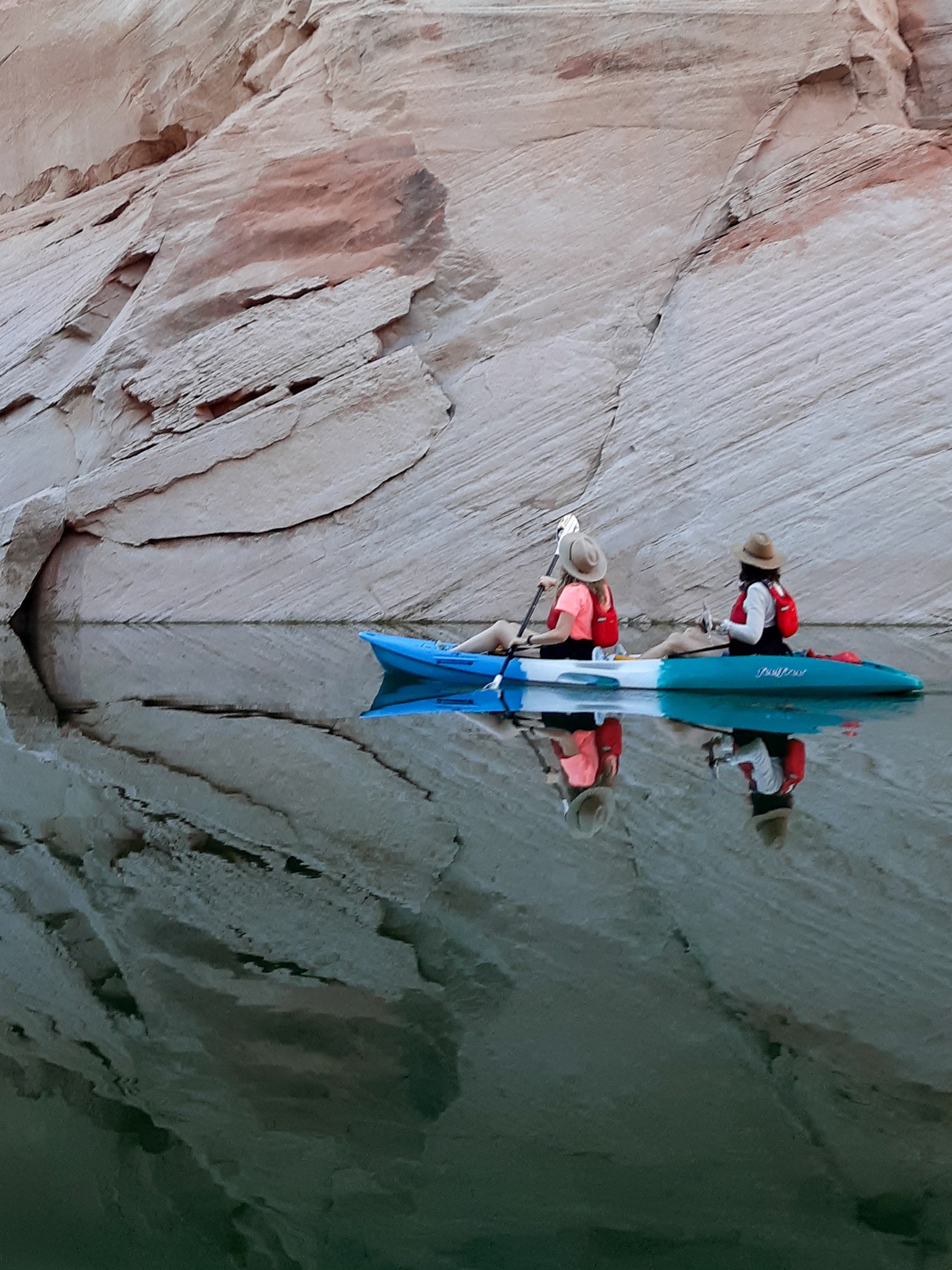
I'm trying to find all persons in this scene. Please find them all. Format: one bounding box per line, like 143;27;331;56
592;531;798;661
652;717;806;852
422;532;619;661
455;711;624;840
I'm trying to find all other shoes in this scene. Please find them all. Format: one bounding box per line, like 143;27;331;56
435;639;442;649
421;643;430;648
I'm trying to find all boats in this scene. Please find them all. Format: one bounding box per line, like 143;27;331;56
356;629;925;698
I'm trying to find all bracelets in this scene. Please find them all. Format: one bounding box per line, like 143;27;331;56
529;726;534;737
527;635;533;646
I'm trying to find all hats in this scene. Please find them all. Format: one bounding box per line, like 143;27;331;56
559;533;607;582
567;786;615;841
742;809;795;850
729;532;786;570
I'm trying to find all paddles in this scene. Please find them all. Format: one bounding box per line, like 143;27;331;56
695;600;714;635
480;512;580;698
498;690;570;823
708;734;720;779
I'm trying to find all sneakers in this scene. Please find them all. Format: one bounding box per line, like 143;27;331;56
614;644;628;658
592;646;611;661
